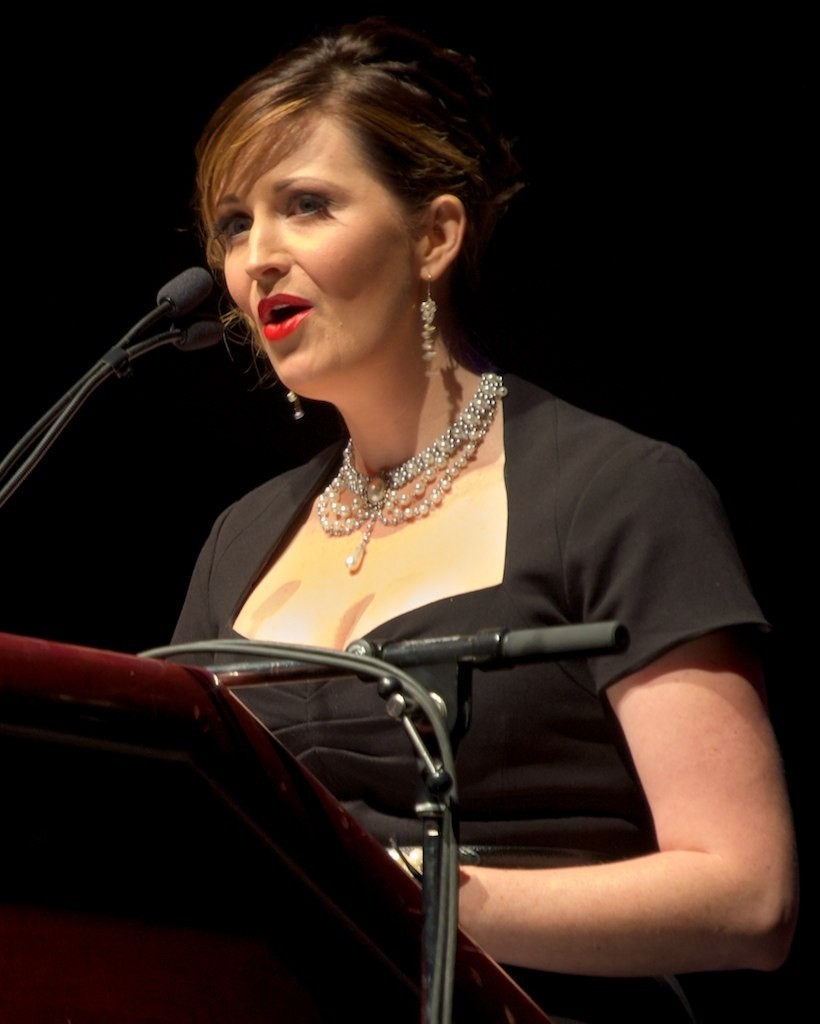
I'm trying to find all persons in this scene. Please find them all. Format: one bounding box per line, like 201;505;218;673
159;31;795;1024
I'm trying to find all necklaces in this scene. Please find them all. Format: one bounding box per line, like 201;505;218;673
315;368;508;571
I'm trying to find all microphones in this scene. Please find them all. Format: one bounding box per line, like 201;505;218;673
0;268;225;509
